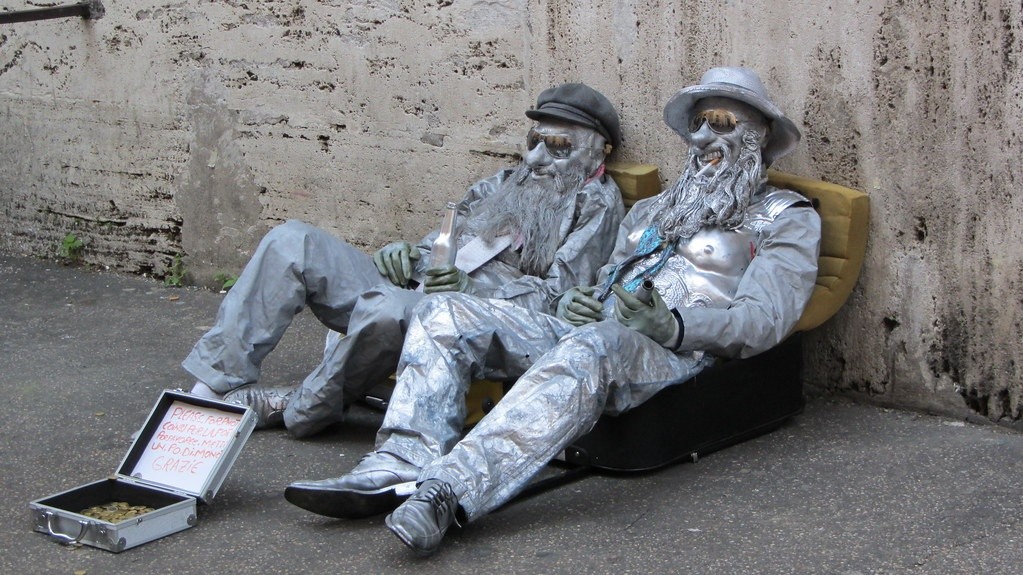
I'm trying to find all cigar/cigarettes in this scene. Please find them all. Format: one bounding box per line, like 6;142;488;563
693;157;720;180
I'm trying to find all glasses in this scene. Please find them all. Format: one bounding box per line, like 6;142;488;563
525;129;602;162
686;108;763;135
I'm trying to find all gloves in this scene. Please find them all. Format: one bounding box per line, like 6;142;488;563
374;241;421;286
556;286;605;327
610;284;677;344
423;263;467;296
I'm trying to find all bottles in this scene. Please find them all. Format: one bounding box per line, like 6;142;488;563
633;278;656;304
427;201;459;268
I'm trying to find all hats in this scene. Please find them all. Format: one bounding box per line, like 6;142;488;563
662;67;803;166
525;84;621;149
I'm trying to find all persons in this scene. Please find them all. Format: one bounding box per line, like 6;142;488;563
129;82;625;448
284;65;824;556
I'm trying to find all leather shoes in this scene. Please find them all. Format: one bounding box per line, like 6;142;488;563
220;383;311;431
284;448;443;520
129;391;225;449
385;477;463;555
